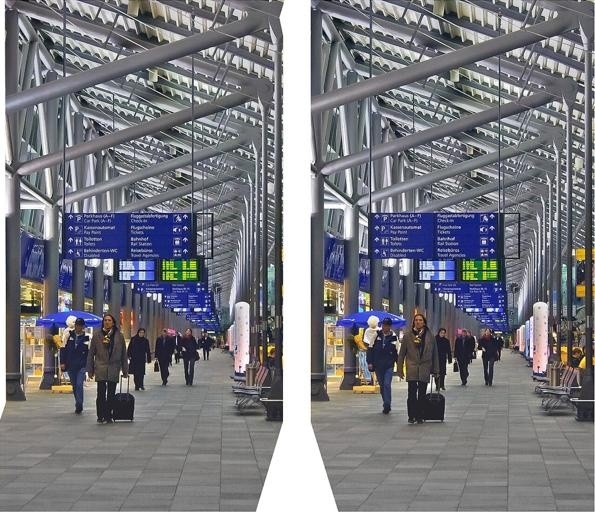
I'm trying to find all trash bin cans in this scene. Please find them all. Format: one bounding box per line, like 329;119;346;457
246;367;256;386
546;364;559;386
515;346;519;351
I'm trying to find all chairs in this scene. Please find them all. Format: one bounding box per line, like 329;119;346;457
531;359;579;415
228;361;270;414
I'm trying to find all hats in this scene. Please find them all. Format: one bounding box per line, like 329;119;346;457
383;317;392;324
74;318;84;324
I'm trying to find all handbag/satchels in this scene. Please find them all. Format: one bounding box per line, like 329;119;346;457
194;351;200;361
128;360;133;373
153;358;160;372
453;361;459;372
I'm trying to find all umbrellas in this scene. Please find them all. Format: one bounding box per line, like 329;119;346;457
36;308;103;328
336;311;407;330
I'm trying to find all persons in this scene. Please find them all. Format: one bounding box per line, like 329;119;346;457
61;314;216;424
363;315;504;423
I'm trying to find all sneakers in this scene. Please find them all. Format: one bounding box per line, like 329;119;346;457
74;409;83;414
436;386;446;391
134;386;145;391
162;380;168;386
408;415;423;424
96;416;112;423
382;407;391;414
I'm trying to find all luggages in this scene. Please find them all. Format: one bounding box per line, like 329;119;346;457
423;374;446;423
112;373;135;423
